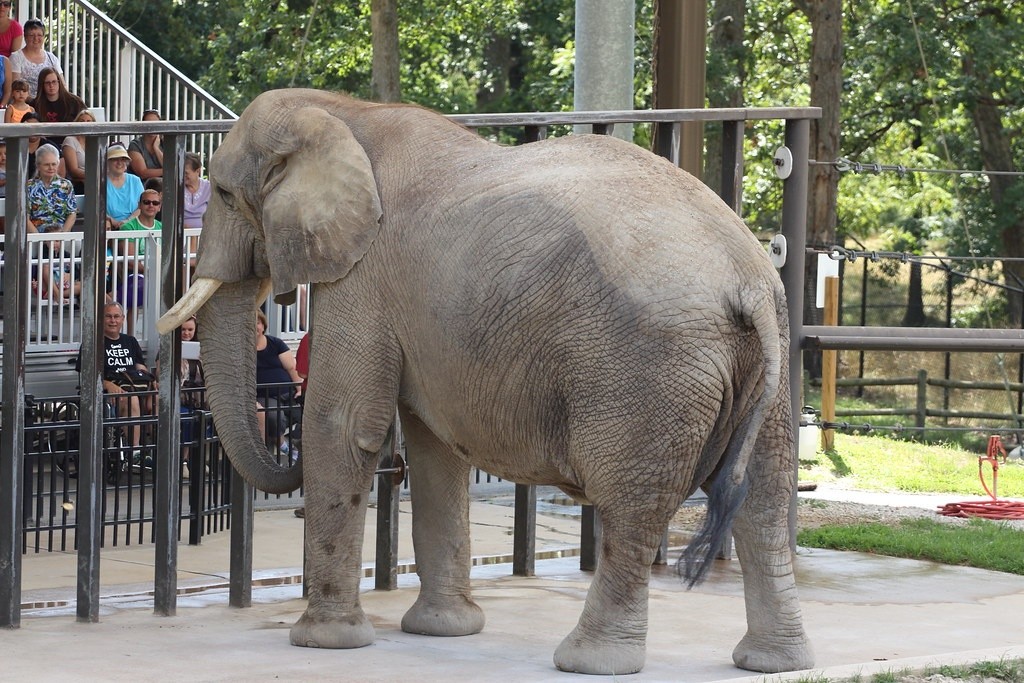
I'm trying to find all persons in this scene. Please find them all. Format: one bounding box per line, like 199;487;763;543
9;18;67;100
118;189;162;272
0;112;66;198
295;333;309;412
154;316;210;478
129;109;163;180
26;143;82;256
183;151;211;228
61;110;96;195
75;301;152;467
256;307;301;468
0;0;23;58
29;67;88;144
5;78;35;123
106;216;113;301
106;142;145;228
0;55;12;109
32;224;81;304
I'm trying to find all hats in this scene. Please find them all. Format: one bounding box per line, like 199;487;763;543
107;144;132;162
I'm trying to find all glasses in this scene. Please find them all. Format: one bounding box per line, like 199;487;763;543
44;80;59;86
145;109;159;114
141;200;160;206
104;313;124;320
0;1;12;8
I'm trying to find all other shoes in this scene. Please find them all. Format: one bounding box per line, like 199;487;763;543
182;464;190;479
295;507;305;518
134;449;153;468
290;450;299;461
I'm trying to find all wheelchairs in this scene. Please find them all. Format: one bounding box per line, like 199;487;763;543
47;357;153;484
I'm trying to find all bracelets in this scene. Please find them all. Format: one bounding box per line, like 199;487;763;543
1;103;6;108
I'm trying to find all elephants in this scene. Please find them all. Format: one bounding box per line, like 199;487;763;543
155;88;816;674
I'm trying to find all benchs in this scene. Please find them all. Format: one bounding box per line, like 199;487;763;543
0;108;107;341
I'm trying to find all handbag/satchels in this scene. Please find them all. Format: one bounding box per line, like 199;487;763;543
108;370;155;392
